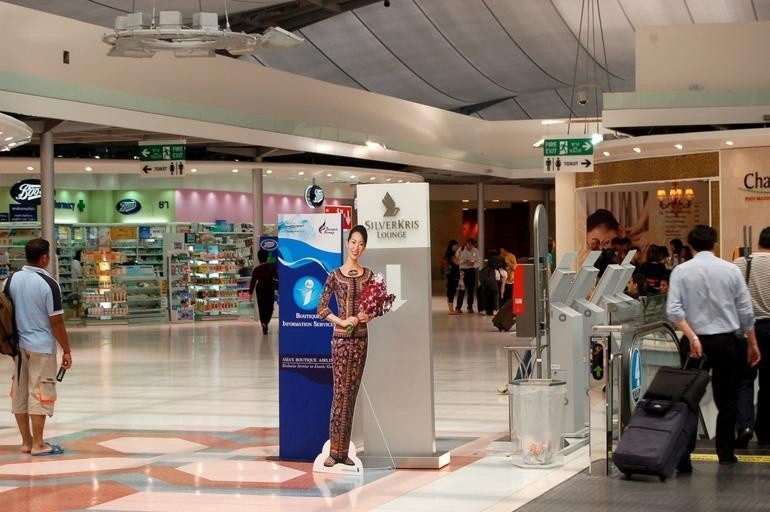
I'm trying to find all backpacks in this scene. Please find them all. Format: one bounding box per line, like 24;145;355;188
440;257;452;274
0;269;20;357
478;267;496;286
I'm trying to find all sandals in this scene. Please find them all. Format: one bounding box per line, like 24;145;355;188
324;453;355;467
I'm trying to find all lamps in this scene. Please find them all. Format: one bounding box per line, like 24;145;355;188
656;156;693;218
534;1;631;152
102;1;304;62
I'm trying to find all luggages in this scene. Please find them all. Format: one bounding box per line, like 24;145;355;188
611;400;698;483
492;299;516;332
476;285;498;313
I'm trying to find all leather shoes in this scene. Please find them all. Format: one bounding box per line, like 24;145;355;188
734;426;753;449
719;455;737;465
676;453;693;472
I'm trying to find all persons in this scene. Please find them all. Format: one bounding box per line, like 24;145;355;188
592;224;695;295
440;237;517;315
1;238;72;456
626;199;652;252
585;208;648;266
664;223;761;474
730;225;769;456
317;226;373;467
71;250;82;293
248;250;278;334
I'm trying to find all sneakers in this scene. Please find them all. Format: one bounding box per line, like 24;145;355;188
261;322;269;335
455;307;463;313
467;306;474;313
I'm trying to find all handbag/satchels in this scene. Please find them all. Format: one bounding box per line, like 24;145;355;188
642;365;712;408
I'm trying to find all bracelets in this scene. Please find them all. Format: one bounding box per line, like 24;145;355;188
687;335;698;340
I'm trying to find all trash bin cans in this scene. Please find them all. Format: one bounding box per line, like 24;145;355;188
508;378;567;469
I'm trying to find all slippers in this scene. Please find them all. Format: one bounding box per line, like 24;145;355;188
448;311;460;316
23;441;64;456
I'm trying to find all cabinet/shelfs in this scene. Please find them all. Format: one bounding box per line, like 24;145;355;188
0;220;279;328
